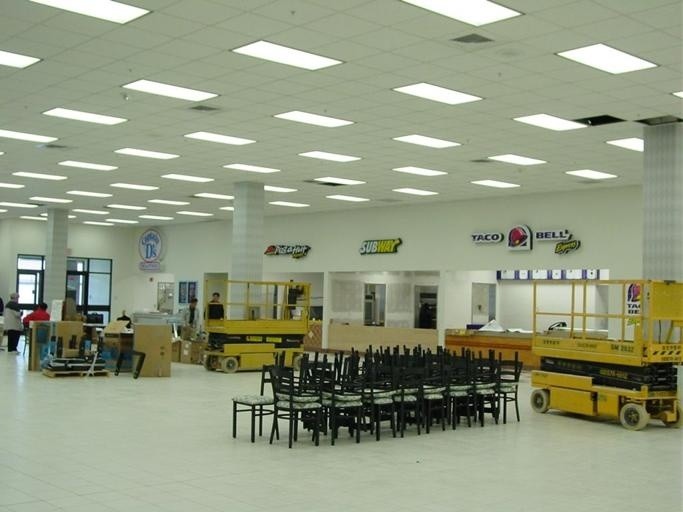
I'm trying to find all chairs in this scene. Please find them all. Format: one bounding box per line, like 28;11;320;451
20;320;30;356
228;342;523;449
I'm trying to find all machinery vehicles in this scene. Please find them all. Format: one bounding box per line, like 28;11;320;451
529;278;682;430
202;278;311;373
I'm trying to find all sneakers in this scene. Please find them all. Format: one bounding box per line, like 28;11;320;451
8;352;20;355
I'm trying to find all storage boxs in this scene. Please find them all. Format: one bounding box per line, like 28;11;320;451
57;321;82;357
180;341;206;363
134;322;171;378
30;321;60;373
171;340;179;361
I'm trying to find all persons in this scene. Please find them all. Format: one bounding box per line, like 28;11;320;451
419;302;433;328
0;296;6;350
3;292;24;355
23;302;50;328
180;298;200;341
204;292;224;320
